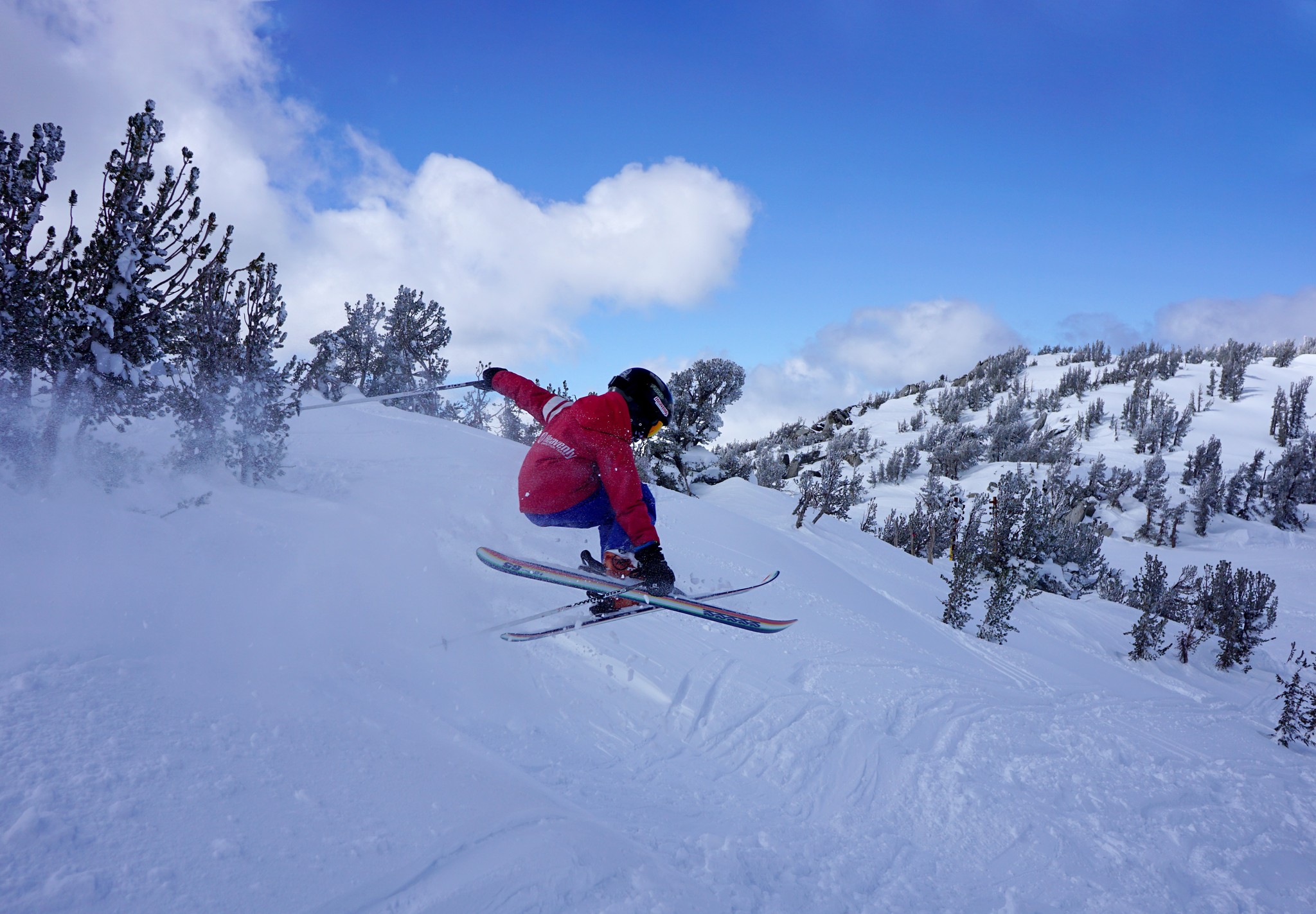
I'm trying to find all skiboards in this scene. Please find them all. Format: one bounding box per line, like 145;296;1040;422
475;546;799;643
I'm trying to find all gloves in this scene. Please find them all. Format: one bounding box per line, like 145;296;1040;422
482;367;507;387
634;544;674;597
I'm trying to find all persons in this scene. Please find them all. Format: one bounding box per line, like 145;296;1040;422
482;367;675;607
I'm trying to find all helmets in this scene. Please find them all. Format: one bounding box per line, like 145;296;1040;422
607;367;675;442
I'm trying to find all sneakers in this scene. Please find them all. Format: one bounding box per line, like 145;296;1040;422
601;550;645;582
604;593;639;610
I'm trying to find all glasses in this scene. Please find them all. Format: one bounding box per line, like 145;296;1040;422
608;375;665;440
